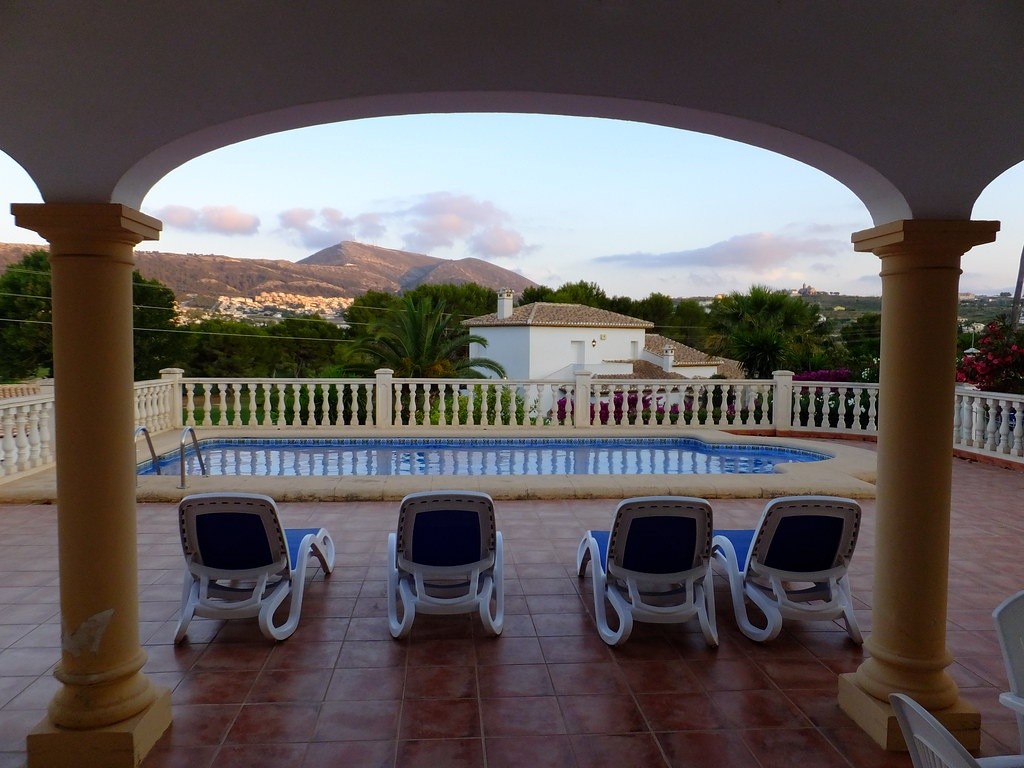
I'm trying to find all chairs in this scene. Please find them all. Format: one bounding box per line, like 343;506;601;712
387;490;504;636
173;492;335;643
889;588;1024;768
711;495;864;646
576;495;719;649
